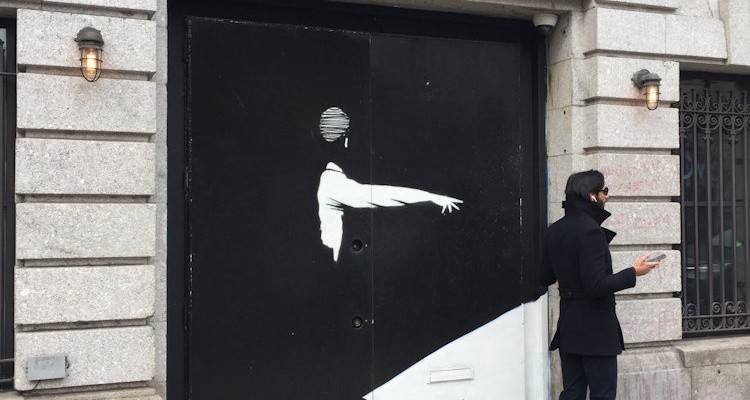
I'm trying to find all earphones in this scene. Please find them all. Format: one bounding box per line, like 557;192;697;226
591;196;597;202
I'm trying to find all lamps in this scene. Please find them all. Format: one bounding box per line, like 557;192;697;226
631;69;662;110
74;28;104;82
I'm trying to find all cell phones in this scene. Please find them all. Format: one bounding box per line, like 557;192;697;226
647;254;667;263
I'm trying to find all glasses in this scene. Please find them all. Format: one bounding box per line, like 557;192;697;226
595;187;609;195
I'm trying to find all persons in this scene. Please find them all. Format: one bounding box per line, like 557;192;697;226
542;170;660;400
